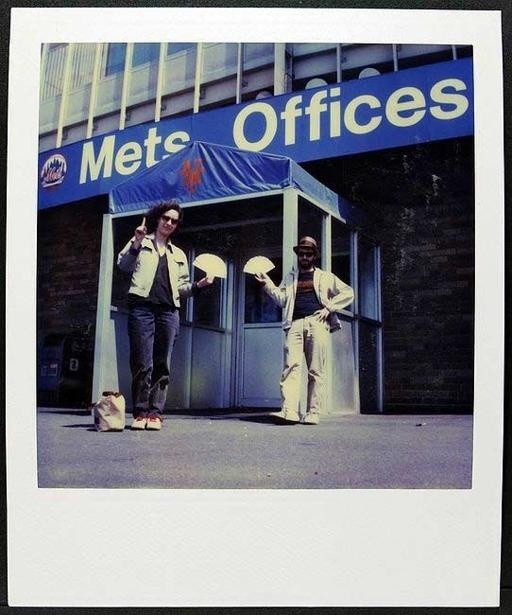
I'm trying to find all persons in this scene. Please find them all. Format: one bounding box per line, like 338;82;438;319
115;200;216;432
253;235;356;423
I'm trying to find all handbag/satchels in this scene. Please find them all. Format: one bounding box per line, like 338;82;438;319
89;390;126;432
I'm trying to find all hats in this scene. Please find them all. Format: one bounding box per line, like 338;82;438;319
163;215;178;225
293;236;319;258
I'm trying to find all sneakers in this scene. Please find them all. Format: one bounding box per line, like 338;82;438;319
131;414;161;430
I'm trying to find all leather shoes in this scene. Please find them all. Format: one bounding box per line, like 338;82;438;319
304;411;320;424
268;409;300;425
